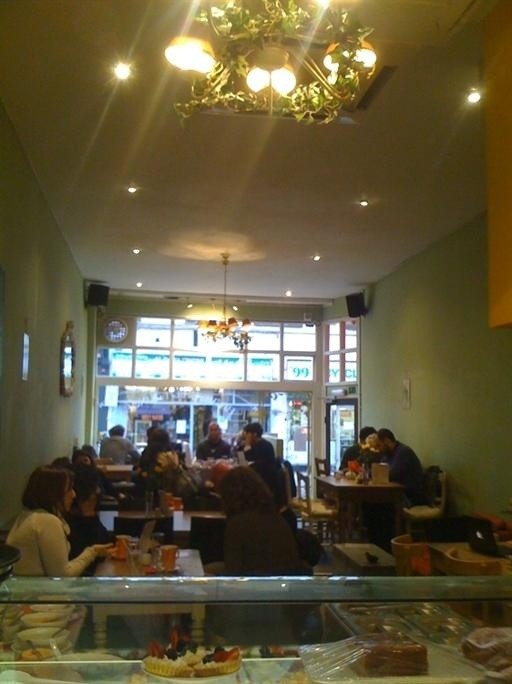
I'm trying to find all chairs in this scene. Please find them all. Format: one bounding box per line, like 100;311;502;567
94;456;505;626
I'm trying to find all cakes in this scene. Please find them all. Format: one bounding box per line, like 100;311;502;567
145;628;242;678
247;649;309;682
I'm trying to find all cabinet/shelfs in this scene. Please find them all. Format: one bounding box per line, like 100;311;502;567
1;576;512;683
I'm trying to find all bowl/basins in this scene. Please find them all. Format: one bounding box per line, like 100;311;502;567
12;602;75;660
0;545;21;585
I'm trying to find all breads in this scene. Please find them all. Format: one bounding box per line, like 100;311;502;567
347;632;430;678
463;626;511;678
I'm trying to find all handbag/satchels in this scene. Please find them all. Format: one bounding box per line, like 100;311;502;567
295;529;325;567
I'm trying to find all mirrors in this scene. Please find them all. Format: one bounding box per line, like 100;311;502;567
59;320;77;393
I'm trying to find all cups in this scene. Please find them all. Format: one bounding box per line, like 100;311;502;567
115;535;131;559
161;545;180;571
164;492;182;511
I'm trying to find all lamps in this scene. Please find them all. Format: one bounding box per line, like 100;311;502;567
196;250;255;350
163;0;377;126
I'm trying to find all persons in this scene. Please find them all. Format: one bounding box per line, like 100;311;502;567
377;428;427;509
339;427;378;473
9;420;313;639
